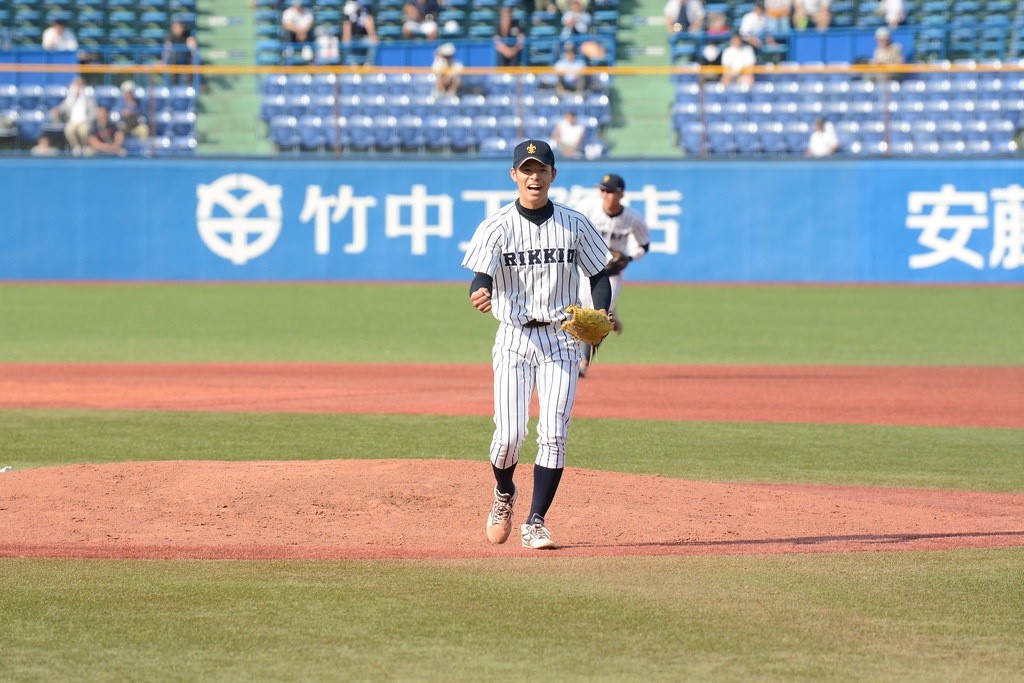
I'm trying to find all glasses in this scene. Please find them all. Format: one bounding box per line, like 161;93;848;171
601;187;614;195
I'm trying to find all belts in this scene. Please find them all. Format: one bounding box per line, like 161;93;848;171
523;318;567;329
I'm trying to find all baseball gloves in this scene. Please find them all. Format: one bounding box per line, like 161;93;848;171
555;304;616;367
606;247;628;276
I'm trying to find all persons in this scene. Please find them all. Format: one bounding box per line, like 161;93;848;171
575;172;651;378
29;16;199;158
281;0;907;97
462;141;614;549
804;115;839;158
549;108;586;162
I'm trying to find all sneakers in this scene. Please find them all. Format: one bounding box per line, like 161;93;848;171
487;480;517;545
520;512;556;550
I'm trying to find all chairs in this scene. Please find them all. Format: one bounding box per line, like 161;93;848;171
668;0;1024;160
251;0;618;162
0;0;202;157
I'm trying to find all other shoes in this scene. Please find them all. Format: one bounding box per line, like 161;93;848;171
577;359;588;378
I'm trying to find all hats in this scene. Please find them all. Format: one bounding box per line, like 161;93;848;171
594;174;625;192
513;139;554;169
